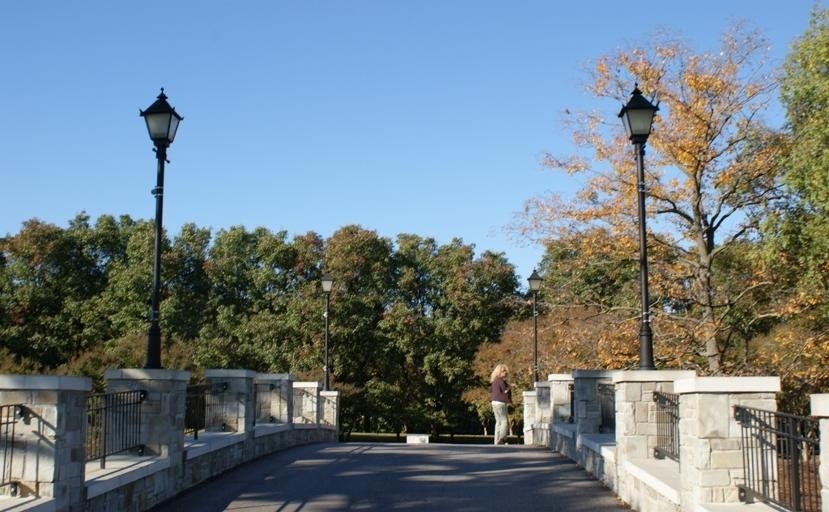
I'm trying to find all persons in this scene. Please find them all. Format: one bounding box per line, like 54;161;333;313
490;363;512;446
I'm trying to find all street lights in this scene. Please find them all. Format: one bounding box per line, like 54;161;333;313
617;79;659;370
320;270;336;391
140;86;185;368
527;267;543;381
